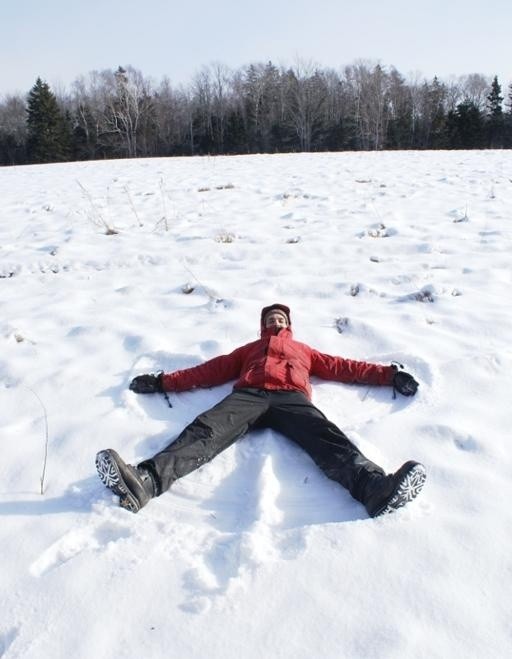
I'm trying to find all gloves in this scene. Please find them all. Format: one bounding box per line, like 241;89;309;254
130;374;163;393
393;371;419;396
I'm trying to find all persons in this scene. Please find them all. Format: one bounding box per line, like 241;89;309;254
95;303;427;520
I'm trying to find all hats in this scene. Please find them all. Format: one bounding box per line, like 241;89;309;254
263;309;289;328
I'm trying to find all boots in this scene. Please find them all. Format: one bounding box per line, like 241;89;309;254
362;461;427;518
96;449;156;513
